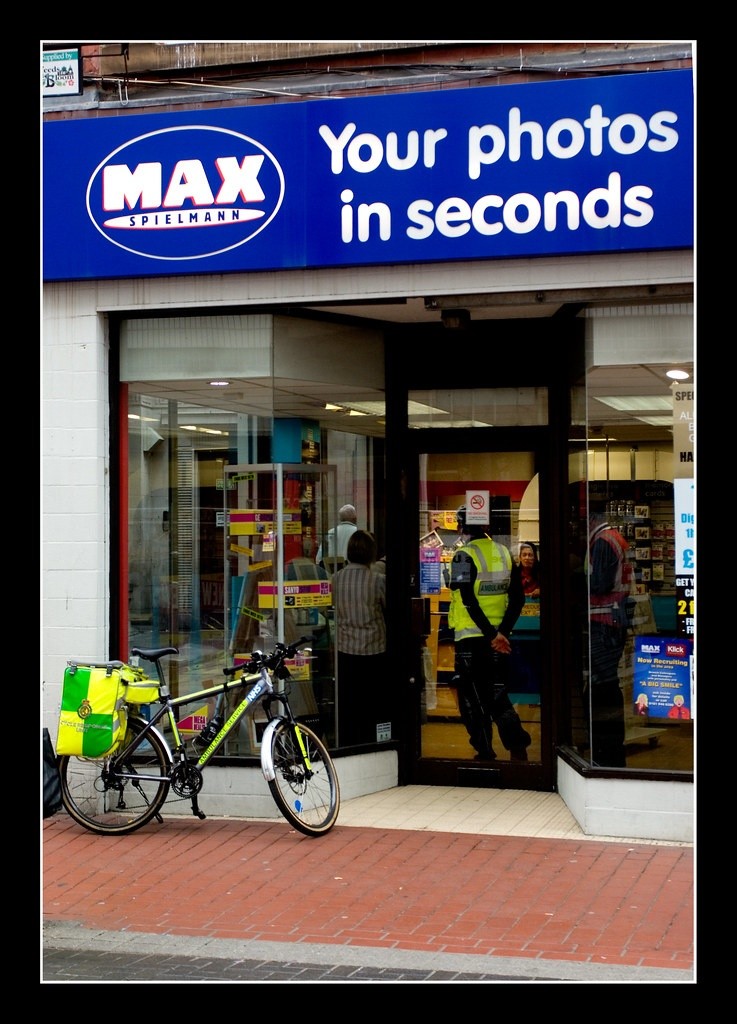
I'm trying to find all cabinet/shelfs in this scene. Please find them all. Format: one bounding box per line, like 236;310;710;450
222;462;339;758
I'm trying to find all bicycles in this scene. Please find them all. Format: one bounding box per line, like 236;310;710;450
58;635;340;837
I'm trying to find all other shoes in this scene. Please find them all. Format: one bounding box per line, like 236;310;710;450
509;748;529;762
592;750;627;768
474;748;498;761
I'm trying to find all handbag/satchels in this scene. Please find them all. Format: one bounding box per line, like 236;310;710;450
44;728;62;817
54;664;148;758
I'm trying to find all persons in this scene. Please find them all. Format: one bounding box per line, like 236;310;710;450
516;541;541;599
316;504;358;564
331;530;386;747
448;507;532;764
582;494;637;768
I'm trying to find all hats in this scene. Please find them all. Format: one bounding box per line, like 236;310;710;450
348;530;377;564
